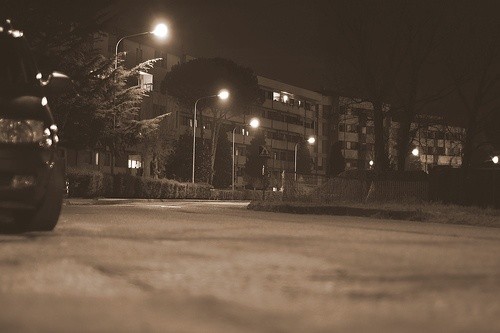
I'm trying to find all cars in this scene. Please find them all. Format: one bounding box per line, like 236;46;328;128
0;42;65;231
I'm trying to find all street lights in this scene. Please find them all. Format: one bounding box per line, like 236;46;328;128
295;136;316;182
191;88;230;185
231;117;260;191
107;20;171;199
